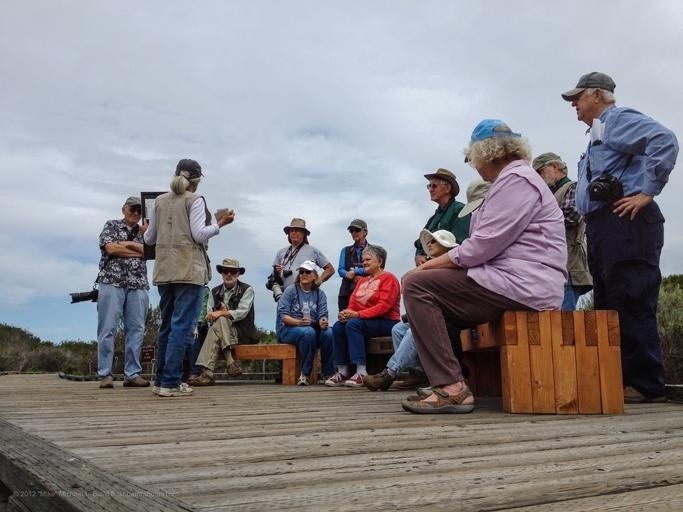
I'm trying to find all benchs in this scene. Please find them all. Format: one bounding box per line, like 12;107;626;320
367;310;625;415
183;344;322;385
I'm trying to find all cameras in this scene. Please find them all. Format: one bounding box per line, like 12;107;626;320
414;238;423;250
265;274;282;302
69;288;98;303
283;269;292;278
589;174;624;204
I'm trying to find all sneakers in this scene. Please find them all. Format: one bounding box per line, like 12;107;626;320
186;373;215;386
363;368;393;391
151;382;194;397
123;376;150;387
227;362;242;377
100;378;114;388
297;372;312;385
316;372;368;386
623;386;665;403
400;369;427;387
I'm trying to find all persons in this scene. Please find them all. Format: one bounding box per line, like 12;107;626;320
270;218;336;384
96;197;150;388
414;168;473;266
362;229;461;392
401;119;568;414
532;153;594;311
561;72;679;404
186;257;259;387
325;219;401;387
140;159;235;397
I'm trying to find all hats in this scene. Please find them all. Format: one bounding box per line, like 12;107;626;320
457;180;491;219
284;218;310;235
348;219;367;228
175;159;201;179
465;119;521;163
532;153;560;170
216;259;245;275
561;72;616;101
420;229;460;259
424;168;459;197
126;196;142;206
296;260;321;277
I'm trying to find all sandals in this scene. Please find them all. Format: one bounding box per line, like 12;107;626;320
402;386;475;414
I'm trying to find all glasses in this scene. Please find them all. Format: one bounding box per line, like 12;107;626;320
427;184;447;189
350;228;361;233
222;268;238;275
427;239;436;245
130;207;141;215
299;270;312;274
